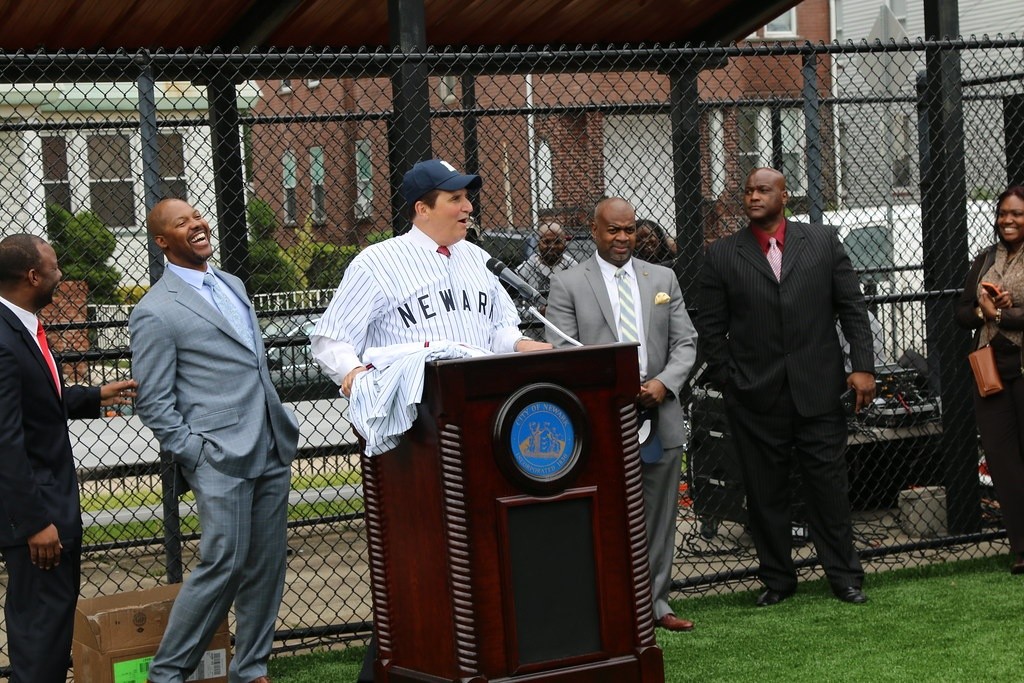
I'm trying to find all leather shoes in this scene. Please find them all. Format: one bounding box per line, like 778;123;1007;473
834;586;868;604
654;613;694;631
756;588;797;607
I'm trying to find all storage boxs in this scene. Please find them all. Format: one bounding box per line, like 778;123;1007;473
74;583;231;683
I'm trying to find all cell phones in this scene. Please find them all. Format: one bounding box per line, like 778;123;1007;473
982;282;1002;301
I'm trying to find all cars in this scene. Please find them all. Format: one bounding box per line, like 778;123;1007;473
260;312;359;402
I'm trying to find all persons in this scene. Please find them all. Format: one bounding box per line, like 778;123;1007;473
695;167;876;607
310;159;553;398
512;222;578;341
545;197;698;630
0;233;137;683
957;187;1024;573
465;216;523;307
129;198;299;683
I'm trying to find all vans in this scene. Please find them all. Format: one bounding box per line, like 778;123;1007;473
783;201;997;364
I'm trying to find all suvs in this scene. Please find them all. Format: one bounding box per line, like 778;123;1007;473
479;223;601;281
666;358;943;526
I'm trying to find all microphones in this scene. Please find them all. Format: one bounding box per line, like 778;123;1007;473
486;257;547;307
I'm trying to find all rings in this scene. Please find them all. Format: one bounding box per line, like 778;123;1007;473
120;392;123;397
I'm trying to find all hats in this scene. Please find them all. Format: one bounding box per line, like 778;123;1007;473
636;402;663;464
403;159;482;207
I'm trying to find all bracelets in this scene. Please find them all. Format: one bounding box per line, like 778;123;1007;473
994;308;1002;324
977;306;983;319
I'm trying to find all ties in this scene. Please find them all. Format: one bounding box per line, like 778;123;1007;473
615;268;643;371
766;237;782;282
37;318;60;396
203;273;256;355
437;246;451;257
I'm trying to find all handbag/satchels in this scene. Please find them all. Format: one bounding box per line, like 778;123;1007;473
966;314;1004;397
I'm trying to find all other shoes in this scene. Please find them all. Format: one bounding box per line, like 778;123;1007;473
248;675;272;683
